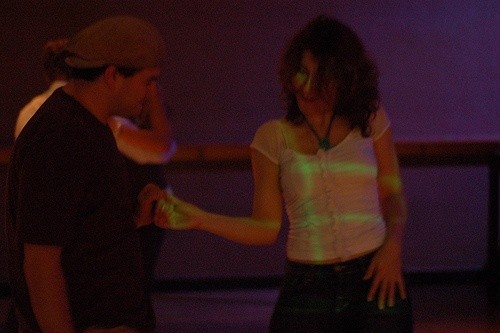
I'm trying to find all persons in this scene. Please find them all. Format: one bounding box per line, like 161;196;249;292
7;16;170;332
154;17;413;333
14;38;174;280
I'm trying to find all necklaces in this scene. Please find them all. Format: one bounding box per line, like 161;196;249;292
300;107;339;153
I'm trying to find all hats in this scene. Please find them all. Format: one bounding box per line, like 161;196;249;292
64;16;169;68
